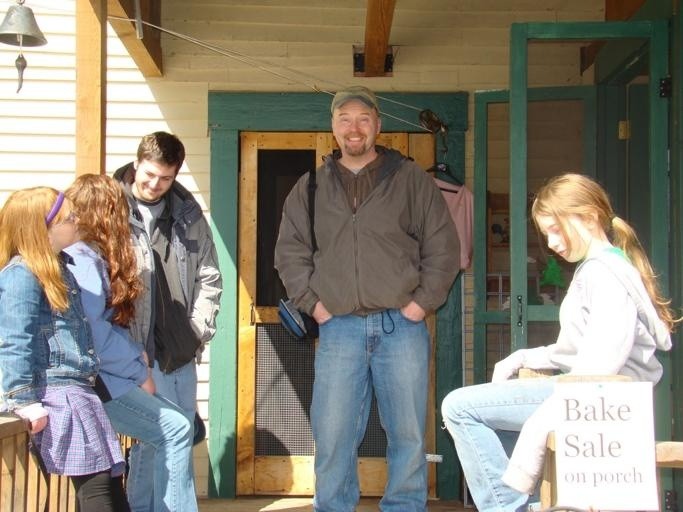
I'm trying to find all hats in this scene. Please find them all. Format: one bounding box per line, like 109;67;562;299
330;85;380;113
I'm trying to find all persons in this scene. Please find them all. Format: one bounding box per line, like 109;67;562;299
274;86;461;512
59;174;201;512
442;174;674;512
110;132;221;512
0;187;130;511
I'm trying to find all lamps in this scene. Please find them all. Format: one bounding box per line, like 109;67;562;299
0;0;48;94
